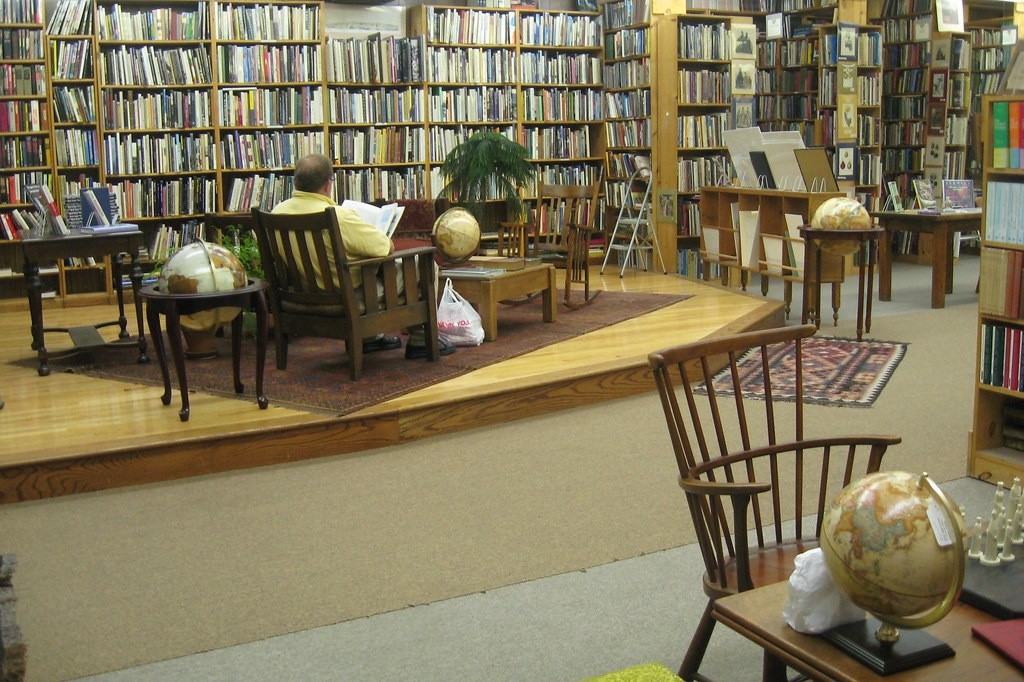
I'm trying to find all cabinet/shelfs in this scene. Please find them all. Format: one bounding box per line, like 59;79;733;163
0;0;603;310
603;0;1024;327
966;93;1024;492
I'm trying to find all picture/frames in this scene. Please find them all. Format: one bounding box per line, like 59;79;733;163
836;143;857;180
655;189;677;223
731;23;757;59
794;147;840;192
837;22;858;61
732;97;757;129
936;0;964;32
731;59;756;95
838;61;857;94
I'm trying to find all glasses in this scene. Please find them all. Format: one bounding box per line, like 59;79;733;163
332;176;335;182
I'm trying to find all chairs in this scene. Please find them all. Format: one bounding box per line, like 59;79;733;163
648;324;902;682
251;206;445;381
494;181;602;311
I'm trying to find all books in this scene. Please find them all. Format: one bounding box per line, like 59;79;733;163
0;0;324;292
677;0;1024;279
325;0;653;275
979;246;1024;451
971;618;1024;666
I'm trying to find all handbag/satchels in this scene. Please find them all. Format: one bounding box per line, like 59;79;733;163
436;276;485;345
782;545;867;635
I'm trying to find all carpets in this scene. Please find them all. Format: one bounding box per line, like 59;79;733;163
5;288;697;415
692;334;907;409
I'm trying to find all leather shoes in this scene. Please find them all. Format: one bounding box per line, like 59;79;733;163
404;338;455;358
345;335;401;354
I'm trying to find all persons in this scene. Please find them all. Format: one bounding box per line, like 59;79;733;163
266;154;456;358
958;477;1024;558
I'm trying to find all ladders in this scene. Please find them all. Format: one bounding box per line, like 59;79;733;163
600;167;668;276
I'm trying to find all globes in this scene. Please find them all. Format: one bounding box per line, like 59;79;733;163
157;240;250;331
818;469;966;677
810;197;872;256
430;206;483;269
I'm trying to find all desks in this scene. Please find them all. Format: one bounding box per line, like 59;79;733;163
868;208;982;309
437;263;558;342
12;226;148;376
711;579;1024;682
